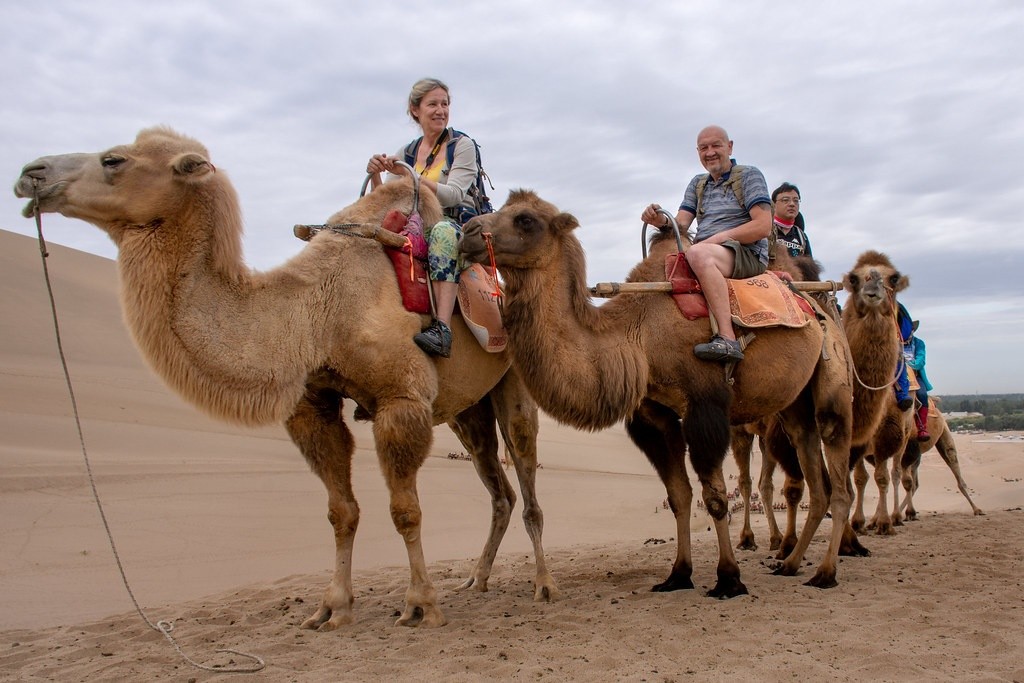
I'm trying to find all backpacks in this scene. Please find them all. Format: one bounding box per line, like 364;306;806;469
405;126;495;225
695;164;778;260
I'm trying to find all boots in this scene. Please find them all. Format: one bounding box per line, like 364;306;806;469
916;405;930;441
895;361;913;411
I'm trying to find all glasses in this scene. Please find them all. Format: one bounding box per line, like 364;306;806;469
776;197;801;204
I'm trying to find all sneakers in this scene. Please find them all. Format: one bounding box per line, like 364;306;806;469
693;334;745;361
413;319;452;358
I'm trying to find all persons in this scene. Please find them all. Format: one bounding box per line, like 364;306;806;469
771;181;819;281
641;126;772;363
895;300;912;412
365;77;478;357
905;318;933;442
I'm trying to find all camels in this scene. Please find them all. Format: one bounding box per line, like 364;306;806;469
457;189;855;601
1;125;561;632
735;250;989;560
